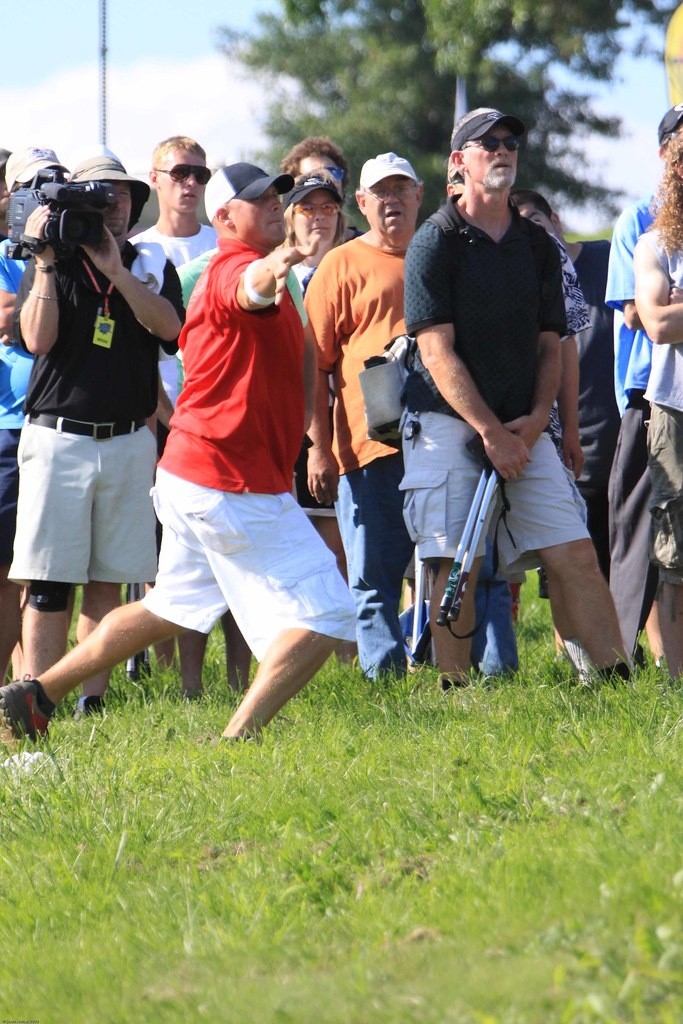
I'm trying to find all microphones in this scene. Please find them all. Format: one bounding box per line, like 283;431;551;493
40;181;69;203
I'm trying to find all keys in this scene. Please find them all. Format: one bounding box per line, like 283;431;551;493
411;421;421;449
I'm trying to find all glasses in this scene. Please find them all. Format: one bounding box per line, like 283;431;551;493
291;200;340;216
369;183;418;201
449;174;464;185
458;136;521;153
324;166;345;180
155;164;211;185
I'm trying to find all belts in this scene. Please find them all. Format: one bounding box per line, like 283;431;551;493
27;413;146;442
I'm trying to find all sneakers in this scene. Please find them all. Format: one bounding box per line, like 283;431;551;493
0;674;55;757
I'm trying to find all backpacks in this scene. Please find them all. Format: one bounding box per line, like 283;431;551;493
359;209;548;453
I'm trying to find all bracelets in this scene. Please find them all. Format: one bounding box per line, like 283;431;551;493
34;264;57;274
28;290;59;300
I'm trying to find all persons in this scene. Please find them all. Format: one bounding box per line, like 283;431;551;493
0;105;683;723
0;162;354;755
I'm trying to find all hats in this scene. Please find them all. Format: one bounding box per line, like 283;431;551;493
0;149;12;169
67;156;150;234
447;155;457;182
5;146;70;194
281;173;341;212
360;153;417;192
204;162;295;223
450;108;525;151
658;103;683;144
